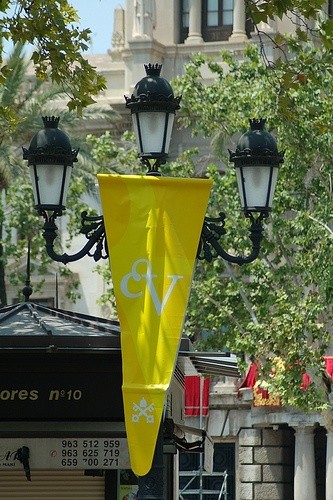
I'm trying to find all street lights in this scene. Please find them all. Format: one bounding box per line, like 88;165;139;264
19;64;287;500
